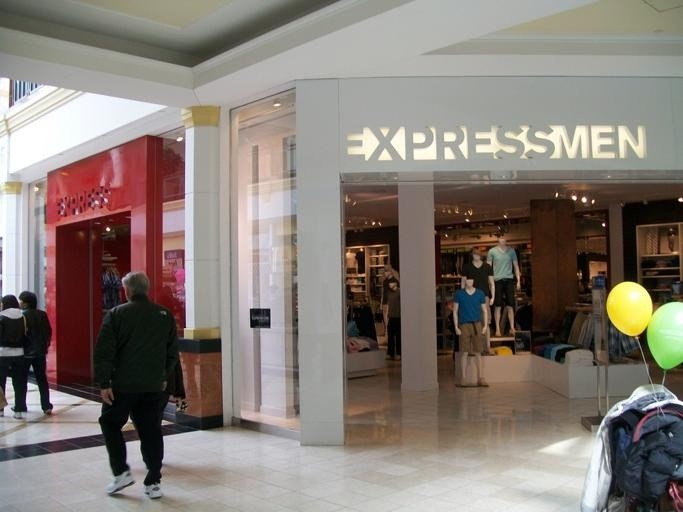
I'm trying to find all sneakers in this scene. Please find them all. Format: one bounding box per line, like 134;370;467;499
460;375;468;386
145;483;161;499
106;470;135;495
0;407;52;419
478;377;488;387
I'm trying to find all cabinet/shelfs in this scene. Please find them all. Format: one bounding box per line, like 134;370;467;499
345;246;365;305
365;244;391;302
435;285;456;354
636;222;683;313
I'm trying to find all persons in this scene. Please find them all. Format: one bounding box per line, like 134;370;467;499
381;237;521;386
0;291;53;419
93;271;186;498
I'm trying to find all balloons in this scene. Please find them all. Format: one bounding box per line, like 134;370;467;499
646;301;683;371
606;281;653;338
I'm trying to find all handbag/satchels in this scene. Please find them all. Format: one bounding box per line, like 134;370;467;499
22;314;33;353
